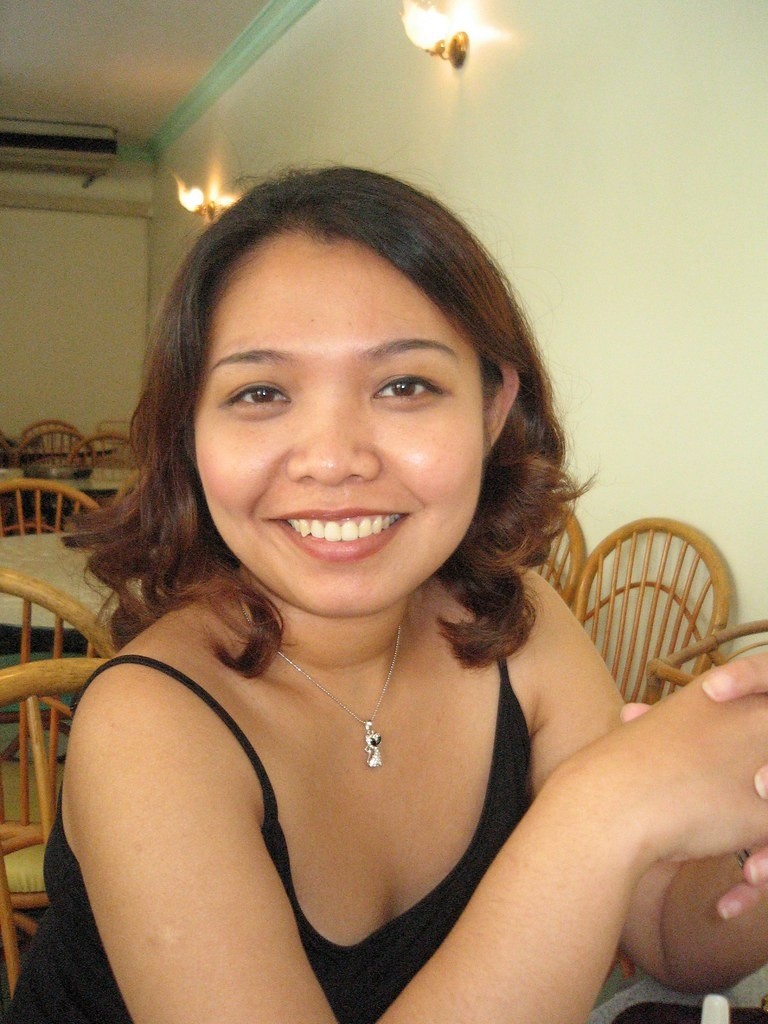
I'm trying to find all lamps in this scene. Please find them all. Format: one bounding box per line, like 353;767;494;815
403;2;471;68
175;182;223;224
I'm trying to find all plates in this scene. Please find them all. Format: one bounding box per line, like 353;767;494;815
24;468;95;481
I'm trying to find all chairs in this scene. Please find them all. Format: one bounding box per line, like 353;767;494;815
0;418;768;999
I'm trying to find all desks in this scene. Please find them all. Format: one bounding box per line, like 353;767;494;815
0;465;138;489
0;531;147;629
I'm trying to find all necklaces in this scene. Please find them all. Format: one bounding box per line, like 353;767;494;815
237;600;402;770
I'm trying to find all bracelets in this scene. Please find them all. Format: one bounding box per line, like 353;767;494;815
734;847;752;868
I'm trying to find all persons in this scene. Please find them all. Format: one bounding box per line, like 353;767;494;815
7;171;768;1024
